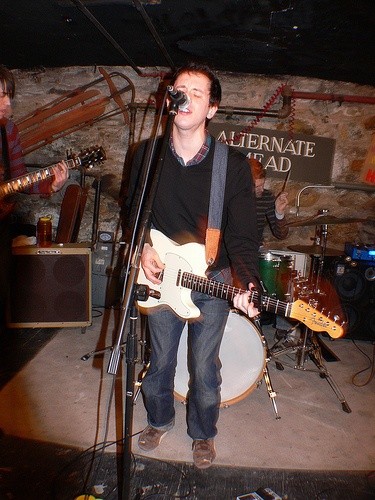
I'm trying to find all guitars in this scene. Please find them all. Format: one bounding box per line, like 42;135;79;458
135;229;346;341
0;145;107;199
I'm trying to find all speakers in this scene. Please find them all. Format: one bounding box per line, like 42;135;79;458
310;256;375;343
0;243;92;328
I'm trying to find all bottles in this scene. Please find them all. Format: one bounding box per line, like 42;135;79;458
37;216;52;247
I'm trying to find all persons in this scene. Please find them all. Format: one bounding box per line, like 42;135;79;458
119;68;260;469
0;69;70;301
247;158;290;254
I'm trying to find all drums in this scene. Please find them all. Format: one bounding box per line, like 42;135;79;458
257;251;294;303
170;264;267;409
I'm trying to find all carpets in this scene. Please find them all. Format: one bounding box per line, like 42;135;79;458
0;306;375;473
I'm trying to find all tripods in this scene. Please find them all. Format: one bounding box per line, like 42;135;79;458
81;306;151;363
268;238;352;414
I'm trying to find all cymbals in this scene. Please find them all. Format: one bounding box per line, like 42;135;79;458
286;244;345;256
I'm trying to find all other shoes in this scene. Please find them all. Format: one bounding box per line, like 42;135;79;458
138;425;168;451
191;438;216;469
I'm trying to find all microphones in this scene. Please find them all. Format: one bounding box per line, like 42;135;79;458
168;86;190;107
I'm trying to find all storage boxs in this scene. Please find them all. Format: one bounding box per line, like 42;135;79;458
345;243;375;261
